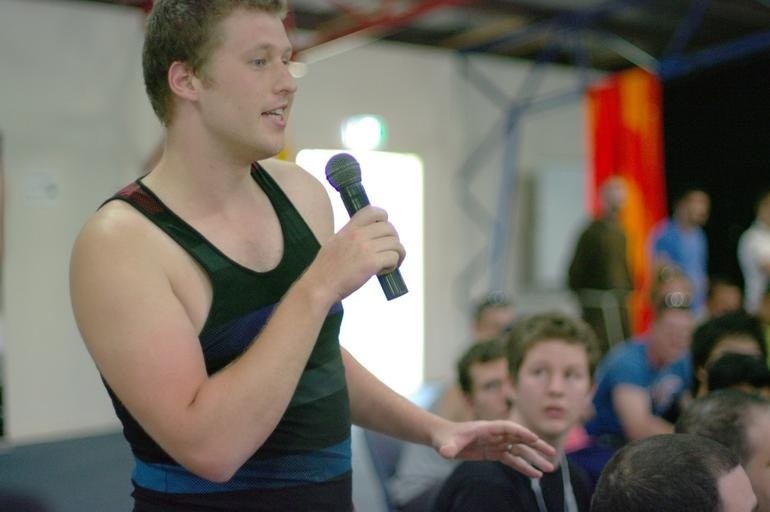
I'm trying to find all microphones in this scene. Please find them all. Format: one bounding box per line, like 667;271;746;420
324;152;409;301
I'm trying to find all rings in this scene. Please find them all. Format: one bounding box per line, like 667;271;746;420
507;443;513;453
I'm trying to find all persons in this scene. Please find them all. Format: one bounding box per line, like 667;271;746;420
566;176;638;357
69;0;556;512
652;190;711;310
399;268;769;512
737;192;770;314
